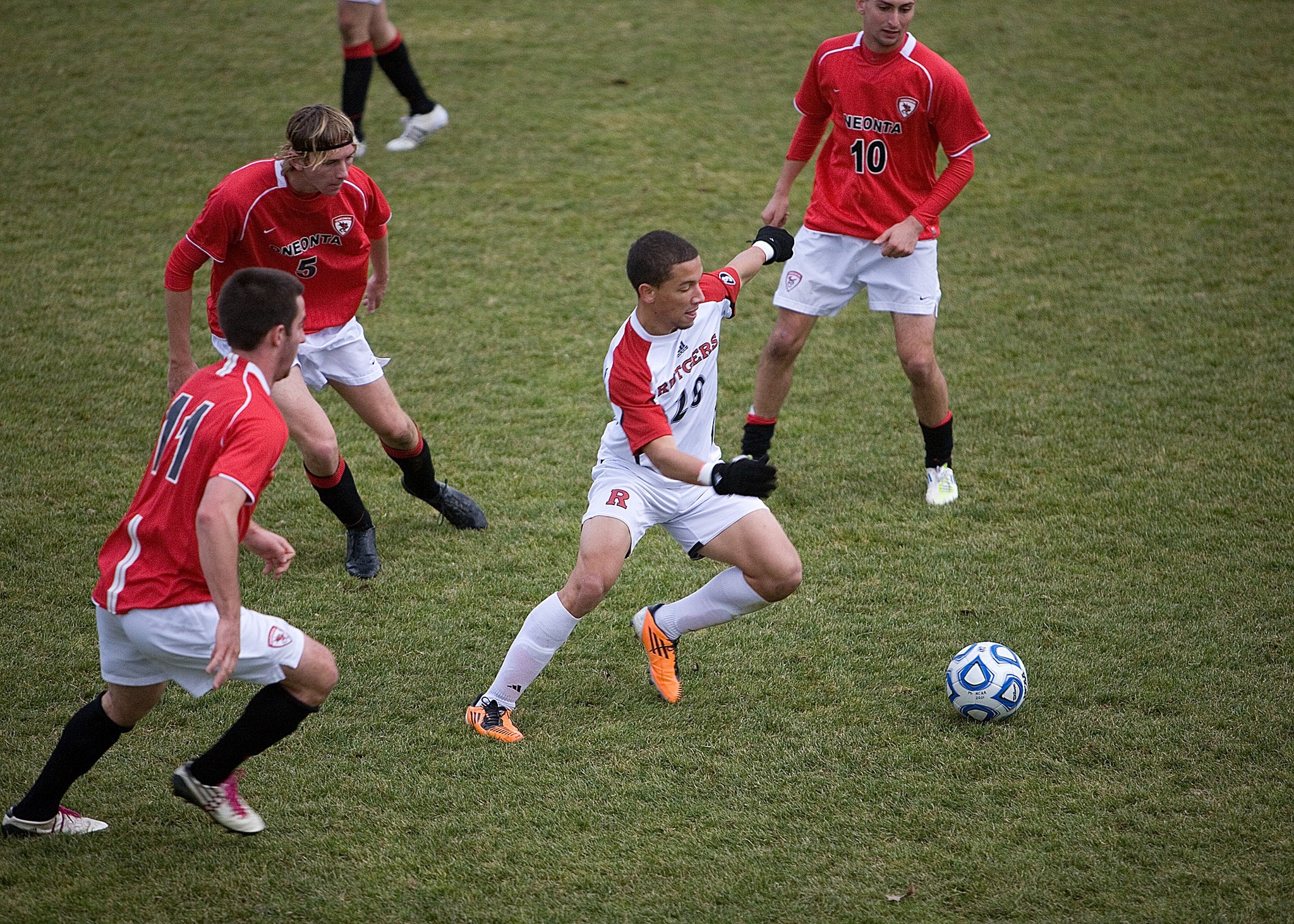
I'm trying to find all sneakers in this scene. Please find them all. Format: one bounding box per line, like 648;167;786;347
347;104;451;158
464;692;525;743
401;473;489;532
1;806;108;838
925;464;958;505
172;759;266;835
345;522;380;580
630;604;684;704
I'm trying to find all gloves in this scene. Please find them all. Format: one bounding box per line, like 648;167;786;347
748;226;797;266
697;456;779;500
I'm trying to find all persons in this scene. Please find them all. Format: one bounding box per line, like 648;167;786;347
160;104;487;580
335;0;448;163
2;265;340;838
460;222;807;742
737;0;995;508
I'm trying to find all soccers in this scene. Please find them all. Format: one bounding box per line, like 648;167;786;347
945;641;1028;723
